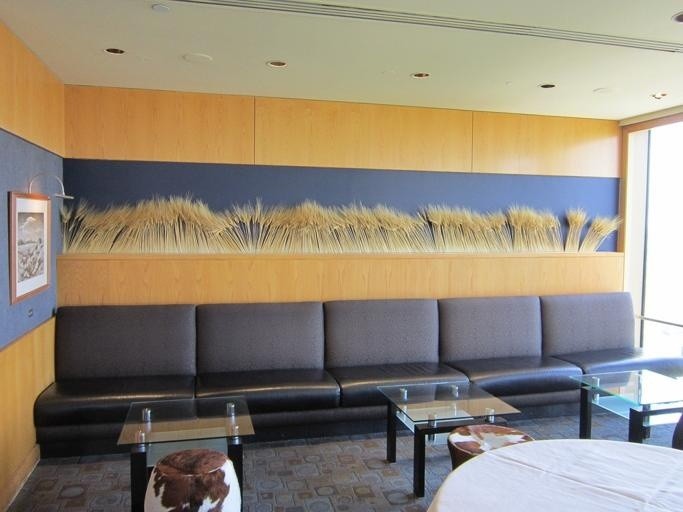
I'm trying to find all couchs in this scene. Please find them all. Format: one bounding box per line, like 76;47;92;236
34;293;683;463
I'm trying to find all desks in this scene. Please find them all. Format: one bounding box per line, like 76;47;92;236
430;440;683;511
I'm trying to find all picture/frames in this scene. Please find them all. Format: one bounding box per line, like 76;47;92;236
7;191;51;303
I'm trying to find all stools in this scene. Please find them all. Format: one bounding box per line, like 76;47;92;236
443;422;533;469
143;450;241;511
